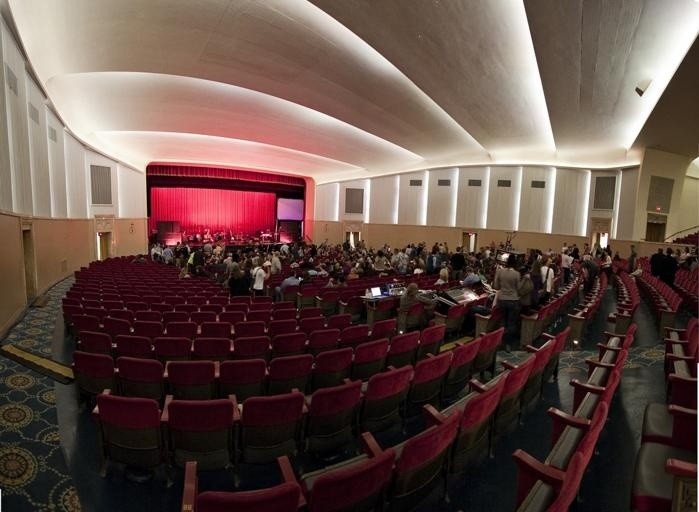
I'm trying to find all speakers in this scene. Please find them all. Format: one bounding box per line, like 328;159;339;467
156;221;182;246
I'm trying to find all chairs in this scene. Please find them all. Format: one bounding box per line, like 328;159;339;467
61;234;696;512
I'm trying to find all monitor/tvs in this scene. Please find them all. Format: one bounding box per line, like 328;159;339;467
370;286;382;297
